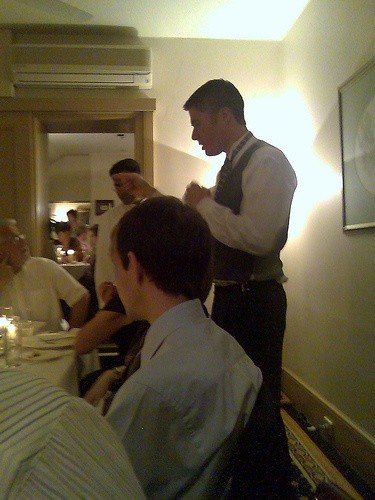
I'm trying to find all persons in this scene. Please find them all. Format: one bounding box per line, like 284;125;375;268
0;218;90;328
49;210;96;321
74;159;149;417
0;368;146;500
96;196;262;500
182;79;296;500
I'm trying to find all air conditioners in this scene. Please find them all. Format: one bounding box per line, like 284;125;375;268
7;43;154;90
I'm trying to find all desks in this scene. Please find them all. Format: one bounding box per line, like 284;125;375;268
16;327;101;397
57;262;91;280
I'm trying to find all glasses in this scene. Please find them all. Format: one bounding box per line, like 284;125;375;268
0;232;25;250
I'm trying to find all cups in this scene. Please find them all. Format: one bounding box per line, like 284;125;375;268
0;306;14;321
3;327;22;368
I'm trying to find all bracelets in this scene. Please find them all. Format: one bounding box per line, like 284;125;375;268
115;368;121;378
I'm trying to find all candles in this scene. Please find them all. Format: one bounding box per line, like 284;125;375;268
0;317;23;367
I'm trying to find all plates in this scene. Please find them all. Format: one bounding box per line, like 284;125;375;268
24;350;61;361
23;334;77;349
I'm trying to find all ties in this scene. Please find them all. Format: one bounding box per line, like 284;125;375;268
218;156;234;190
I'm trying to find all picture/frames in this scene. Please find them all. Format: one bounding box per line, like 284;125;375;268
336;53;375;233
